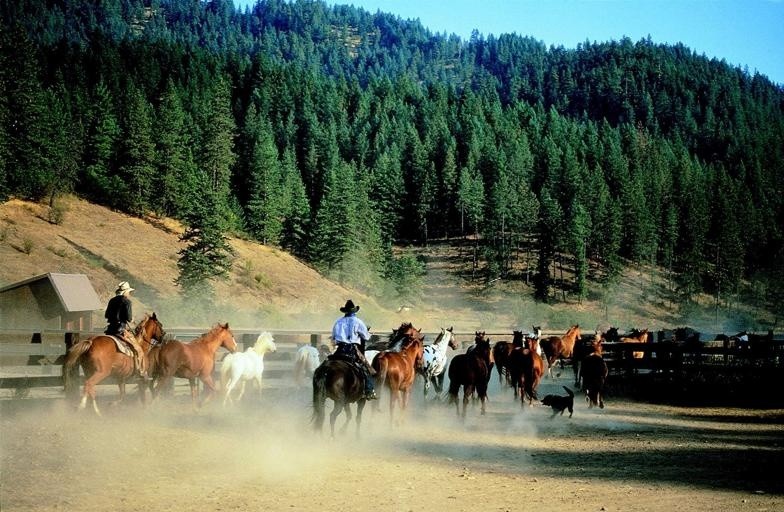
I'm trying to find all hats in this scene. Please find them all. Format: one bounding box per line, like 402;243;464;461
116;281;135;296
340;300;359;312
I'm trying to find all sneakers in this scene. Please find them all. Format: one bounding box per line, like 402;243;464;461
135;370;153;381
367;389;380;399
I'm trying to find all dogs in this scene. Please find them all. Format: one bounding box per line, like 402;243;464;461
540;385;575;418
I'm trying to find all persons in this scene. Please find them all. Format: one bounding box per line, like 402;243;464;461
332;299;379;399
104;281;151;379
737;329;749;342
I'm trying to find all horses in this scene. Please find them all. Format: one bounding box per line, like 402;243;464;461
364;322;648;428
306;327;370;443
63;313;167;419
293;343;337;387
148;322;240;416
219;332;277;409
